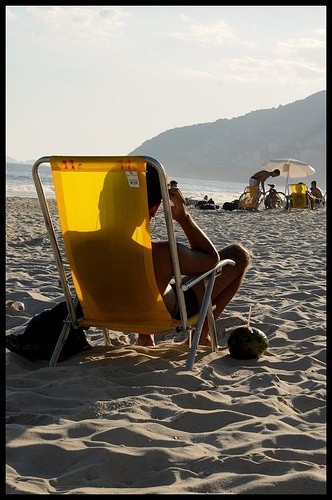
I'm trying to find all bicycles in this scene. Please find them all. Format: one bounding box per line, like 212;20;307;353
238;184;289;211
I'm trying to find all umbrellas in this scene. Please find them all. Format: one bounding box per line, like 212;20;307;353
262;157;317;203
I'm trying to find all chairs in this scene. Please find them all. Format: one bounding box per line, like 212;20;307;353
308;191;327;211
287;182;312;213
33;154;236;373
237;184;264;212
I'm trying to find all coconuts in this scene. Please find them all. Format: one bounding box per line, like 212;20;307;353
228;326;269;360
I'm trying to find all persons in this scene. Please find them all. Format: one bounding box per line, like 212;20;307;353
309;180;324;205
246;168;281;212
290;181;315;208
63;161;250;349
167;180;178;189
